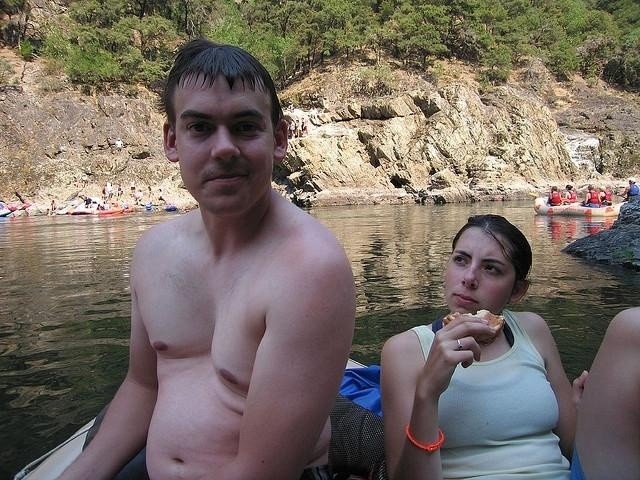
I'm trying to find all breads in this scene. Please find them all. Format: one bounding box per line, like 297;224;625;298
443;309;505;342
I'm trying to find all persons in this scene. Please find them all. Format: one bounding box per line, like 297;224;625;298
548;180;639;207
550;215;613;242
288;106;318;139
115;138;123;153
84;183;162;211
380;214;640;480
53;38;356;480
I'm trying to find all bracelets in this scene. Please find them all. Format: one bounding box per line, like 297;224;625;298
405;423;445;452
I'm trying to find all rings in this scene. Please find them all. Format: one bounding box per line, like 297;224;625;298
457;339;462;351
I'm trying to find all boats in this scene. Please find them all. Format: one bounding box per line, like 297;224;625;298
533;197;628;217
47;202;177;215
0;202;16;216
